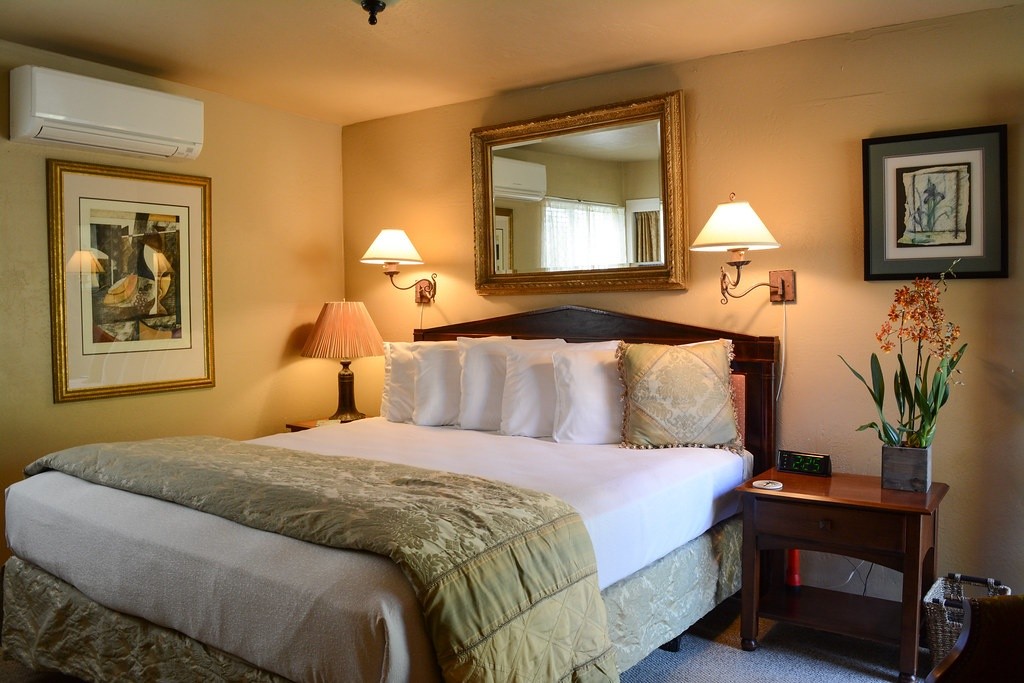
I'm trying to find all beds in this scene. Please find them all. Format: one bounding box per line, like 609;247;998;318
0;304;783;683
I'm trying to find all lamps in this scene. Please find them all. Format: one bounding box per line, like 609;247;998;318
302;299;386;420
694;190;798;303
360;229;437;303
346;0;396;25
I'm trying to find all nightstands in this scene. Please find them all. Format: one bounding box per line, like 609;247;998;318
733;466;949;683
286;417;375;432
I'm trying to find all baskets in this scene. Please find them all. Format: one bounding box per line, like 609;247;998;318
923;573;1012;670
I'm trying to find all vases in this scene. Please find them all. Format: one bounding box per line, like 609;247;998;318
878;440;939;492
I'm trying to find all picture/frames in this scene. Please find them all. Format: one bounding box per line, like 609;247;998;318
47;157;218;402
859;123;1012;281
491;206;516;277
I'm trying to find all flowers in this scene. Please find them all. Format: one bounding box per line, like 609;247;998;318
837;282;967;445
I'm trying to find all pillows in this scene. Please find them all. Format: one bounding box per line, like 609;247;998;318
456;335;511;434
551;339;627;446
377;339;420;423
498;336;567;439
615;338;743;453
406;340;463;427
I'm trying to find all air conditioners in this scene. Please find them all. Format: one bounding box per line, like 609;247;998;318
7;64;204;162
492;155;548;202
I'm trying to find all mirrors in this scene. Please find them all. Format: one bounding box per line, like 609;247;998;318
469;88;691;297
